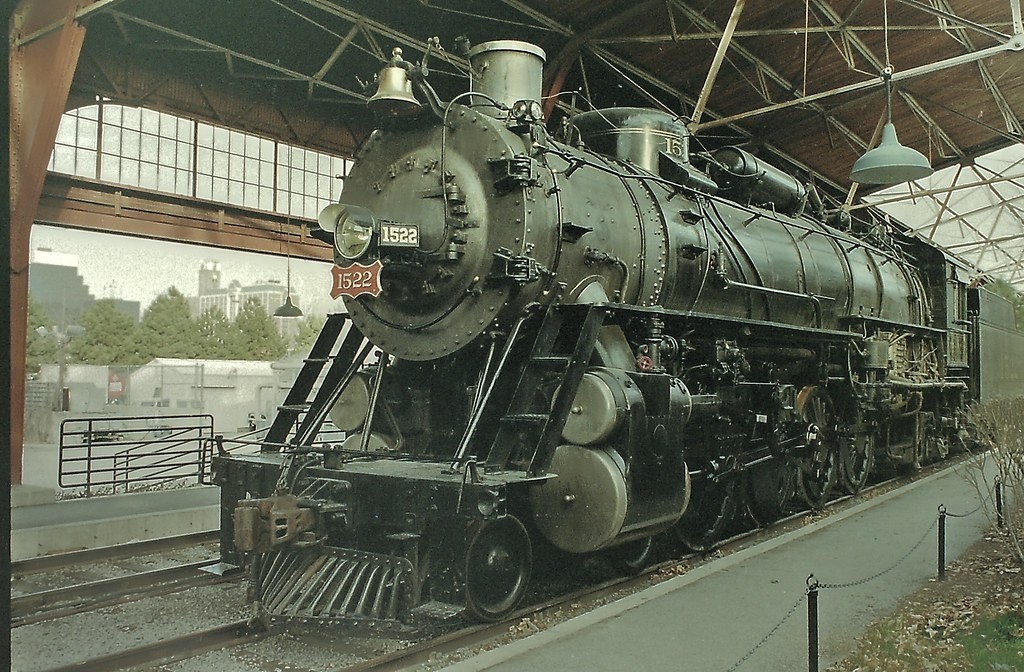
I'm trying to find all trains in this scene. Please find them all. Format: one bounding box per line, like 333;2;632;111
198;39;1024;631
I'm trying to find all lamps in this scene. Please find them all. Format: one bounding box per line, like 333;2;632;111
848;0;934;186
273;125;304;319
